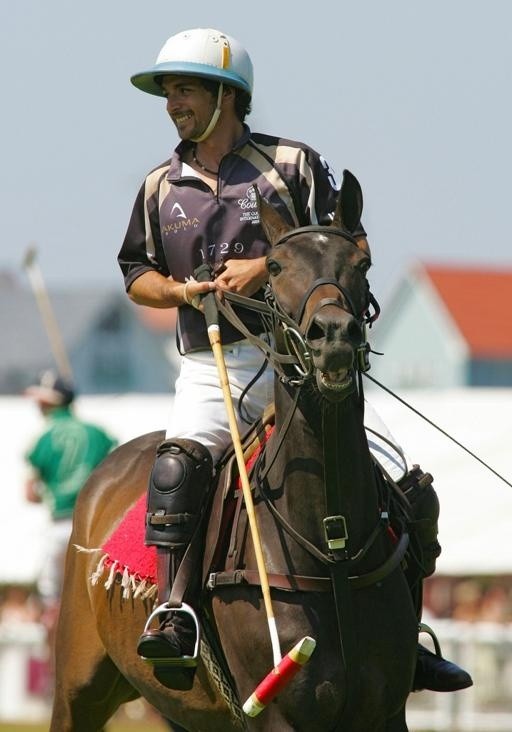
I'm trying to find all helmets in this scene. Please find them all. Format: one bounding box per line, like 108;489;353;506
130;26;256;93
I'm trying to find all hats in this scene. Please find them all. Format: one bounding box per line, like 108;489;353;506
23;374;80;409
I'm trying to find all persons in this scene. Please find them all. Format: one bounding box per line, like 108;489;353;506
110;17;475;694
18;365;120;637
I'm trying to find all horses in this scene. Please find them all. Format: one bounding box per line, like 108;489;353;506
45;168;419;732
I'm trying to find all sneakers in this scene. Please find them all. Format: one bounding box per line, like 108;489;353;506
136;621;198;692
413;639;474;692
